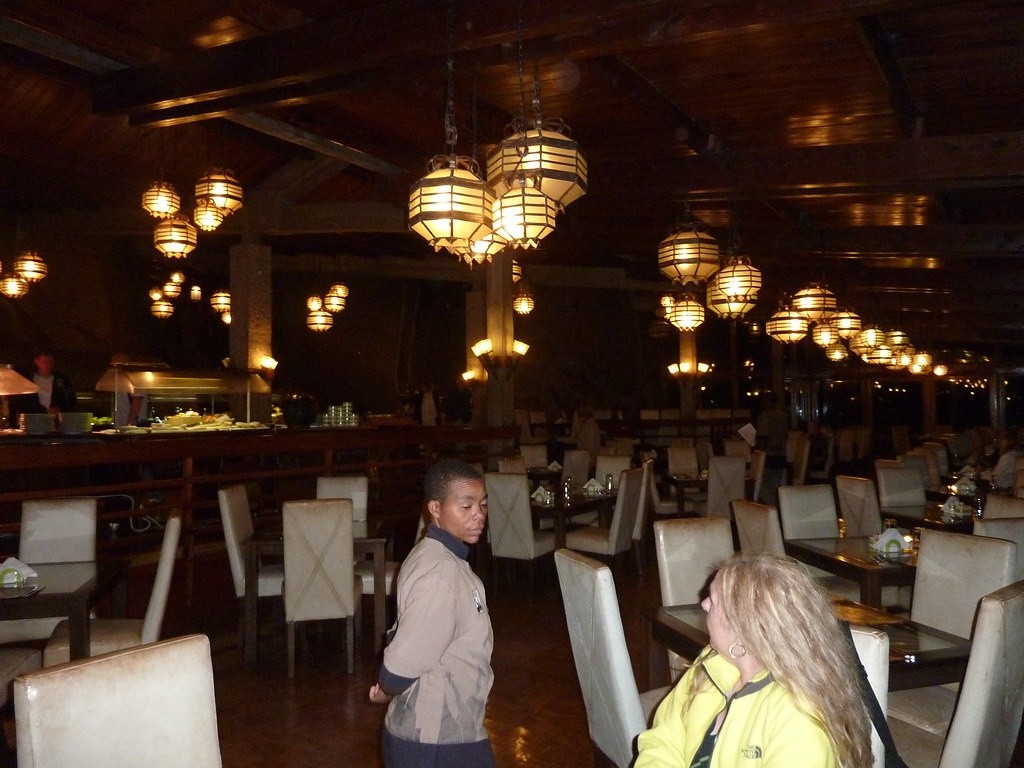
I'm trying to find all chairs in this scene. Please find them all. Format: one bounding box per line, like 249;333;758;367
0;405;1024;768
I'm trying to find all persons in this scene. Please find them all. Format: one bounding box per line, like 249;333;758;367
805;418;827;482
980;438;1024;493
414;379;475;427
18;345;78;416
369;459;497;768
112;351;148;428
629;552;878;768
545;407;602;481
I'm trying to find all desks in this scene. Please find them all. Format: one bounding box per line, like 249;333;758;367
0;421;1024;768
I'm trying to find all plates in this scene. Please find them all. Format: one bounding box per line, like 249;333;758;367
20;413;94;435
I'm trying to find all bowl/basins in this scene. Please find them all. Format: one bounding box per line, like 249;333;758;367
167;416;202;426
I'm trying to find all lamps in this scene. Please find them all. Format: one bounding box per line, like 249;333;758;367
142;118;243;260
306;248;349;332
222;357;230;367
260;355;278;380
749;322;762;335
408;0;588;272
658;153;761;333
0;249;48;299
512;253;522;283
668;363;709;383
471;339;497;379
507;340;529;381
767;282;948;376
513;288;534;314
148;269;232;325
